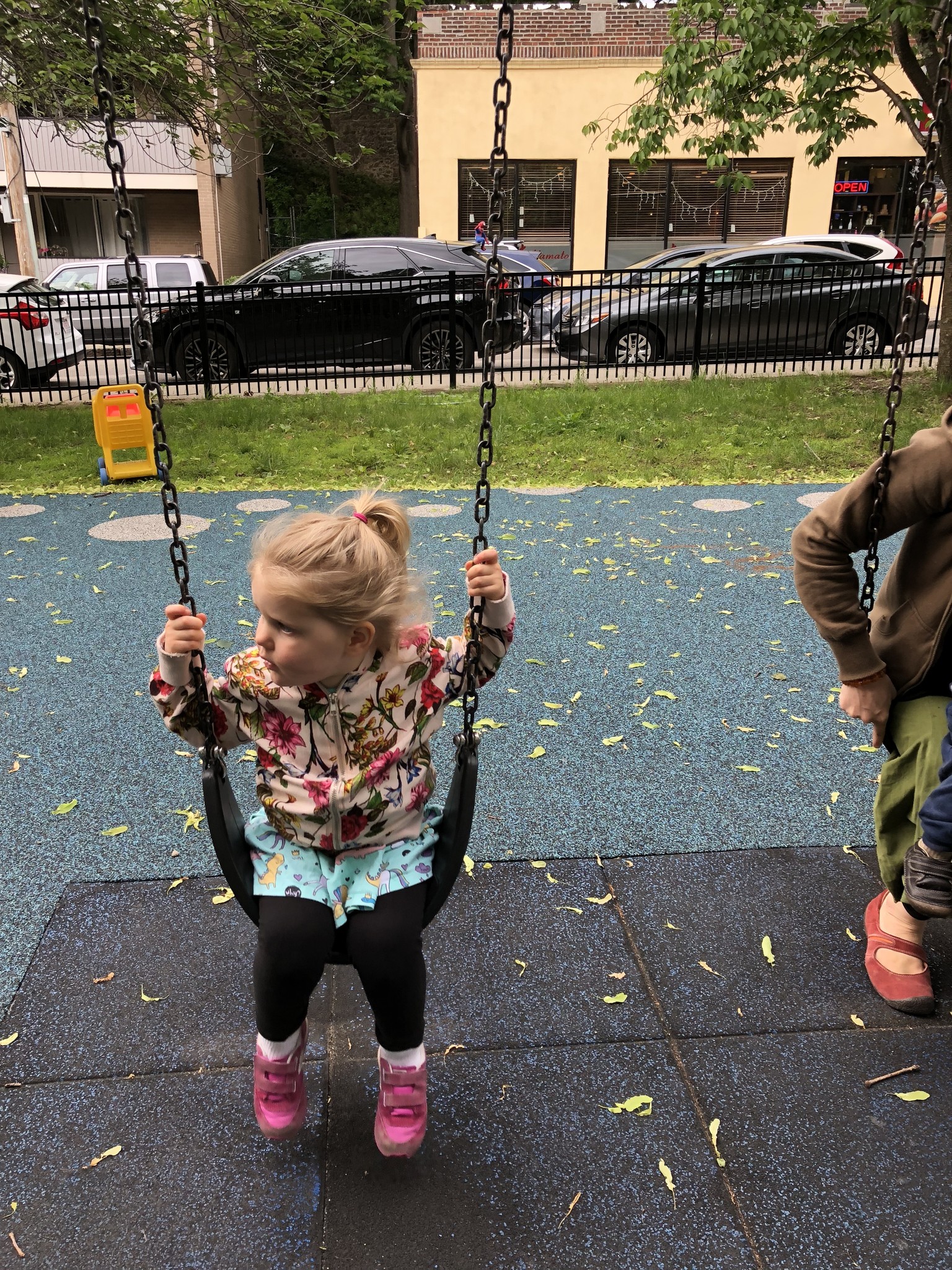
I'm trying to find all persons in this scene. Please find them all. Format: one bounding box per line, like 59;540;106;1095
150;487;513;1159
792;407;951;1010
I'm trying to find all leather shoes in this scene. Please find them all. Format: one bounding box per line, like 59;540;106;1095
904;838;952;917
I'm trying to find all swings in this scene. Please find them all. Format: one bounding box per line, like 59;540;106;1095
858;1;952;763
82;0;516;967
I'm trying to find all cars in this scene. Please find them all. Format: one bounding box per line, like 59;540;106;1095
523;243;750;347
546;244;930;367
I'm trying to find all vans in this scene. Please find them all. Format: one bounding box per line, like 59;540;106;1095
33;255;220;344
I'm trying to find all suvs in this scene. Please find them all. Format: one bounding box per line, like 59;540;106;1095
127;233;534;383
471;240;564;345
0;271;87;394
753;233;907;275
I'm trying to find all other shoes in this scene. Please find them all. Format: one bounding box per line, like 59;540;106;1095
861;889;934;1015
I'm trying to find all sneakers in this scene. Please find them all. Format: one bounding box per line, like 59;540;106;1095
252;1016;310;1140
373;1044;428;1160
